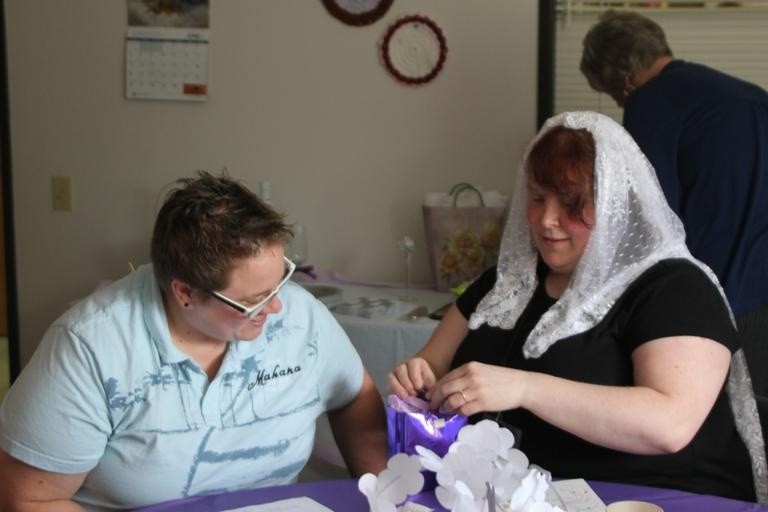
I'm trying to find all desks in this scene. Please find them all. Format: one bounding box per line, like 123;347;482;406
297;285;479;469
125;475;765;512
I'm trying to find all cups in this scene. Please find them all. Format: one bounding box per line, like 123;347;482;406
287;224;307;263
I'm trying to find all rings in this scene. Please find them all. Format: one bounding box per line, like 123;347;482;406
459;390;468;403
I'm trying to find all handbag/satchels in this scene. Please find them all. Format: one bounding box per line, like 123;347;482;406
422;183;505;292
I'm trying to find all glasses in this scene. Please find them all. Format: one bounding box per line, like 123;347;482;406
171;255;297;317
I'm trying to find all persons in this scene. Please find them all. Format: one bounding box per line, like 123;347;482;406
0;166;388;510
577;8;766;397
388;111;766;501
